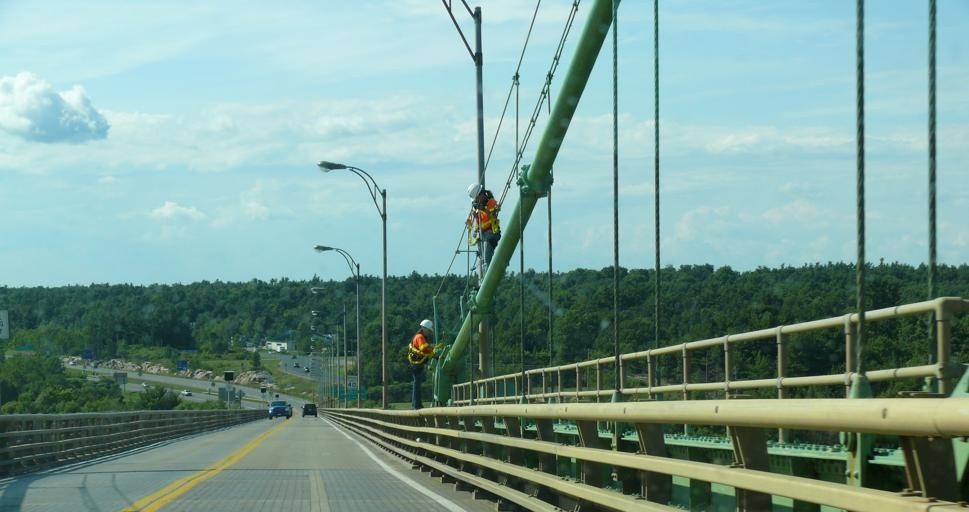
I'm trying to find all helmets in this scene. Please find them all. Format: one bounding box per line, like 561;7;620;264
467;183;483;201
419;319;433;333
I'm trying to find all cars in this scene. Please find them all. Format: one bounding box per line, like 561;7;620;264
301;404;317;418
268;400;292;420
142;382;149;387
181;389;192;396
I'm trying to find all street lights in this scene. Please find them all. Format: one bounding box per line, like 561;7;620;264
311;160;388;410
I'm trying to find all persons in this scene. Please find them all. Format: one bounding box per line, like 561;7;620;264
465;183;501;273
407;319;443;410
